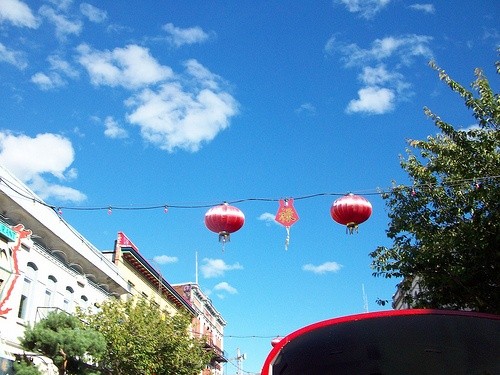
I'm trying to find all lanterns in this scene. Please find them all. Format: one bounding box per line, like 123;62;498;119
204;202;245;252
330;192;372;235
